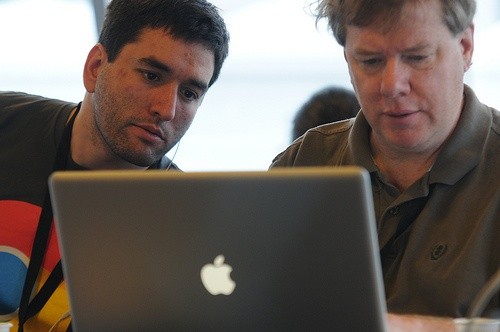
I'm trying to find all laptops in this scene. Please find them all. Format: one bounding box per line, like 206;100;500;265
48;166;387;331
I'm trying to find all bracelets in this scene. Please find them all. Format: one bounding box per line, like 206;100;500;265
452;314;472;332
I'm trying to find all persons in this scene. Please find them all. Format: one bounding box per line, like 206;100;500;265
0;1;237;331
289;87;360;148
261;0;499;330
